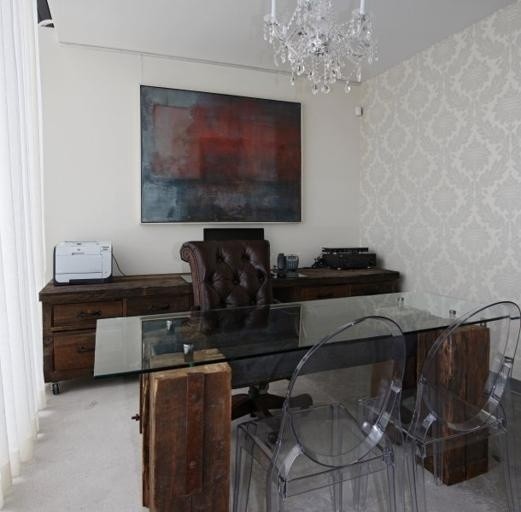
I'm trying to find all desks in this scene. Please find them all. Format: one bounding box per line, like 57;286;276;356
92;292;521;512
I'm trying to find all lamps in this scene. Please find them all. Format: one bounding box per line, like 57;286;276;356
263;1;379;97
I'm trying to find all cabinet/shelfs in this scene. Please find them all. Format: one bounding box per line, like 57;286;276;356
39;267;401;394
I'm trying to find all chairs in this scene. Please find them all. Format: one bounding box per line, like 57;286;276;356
356;301;521;512
180;241;313;420
232;316;417;512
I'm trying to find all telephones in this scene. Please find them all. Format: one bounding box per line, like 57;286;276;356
278;253;299;271
